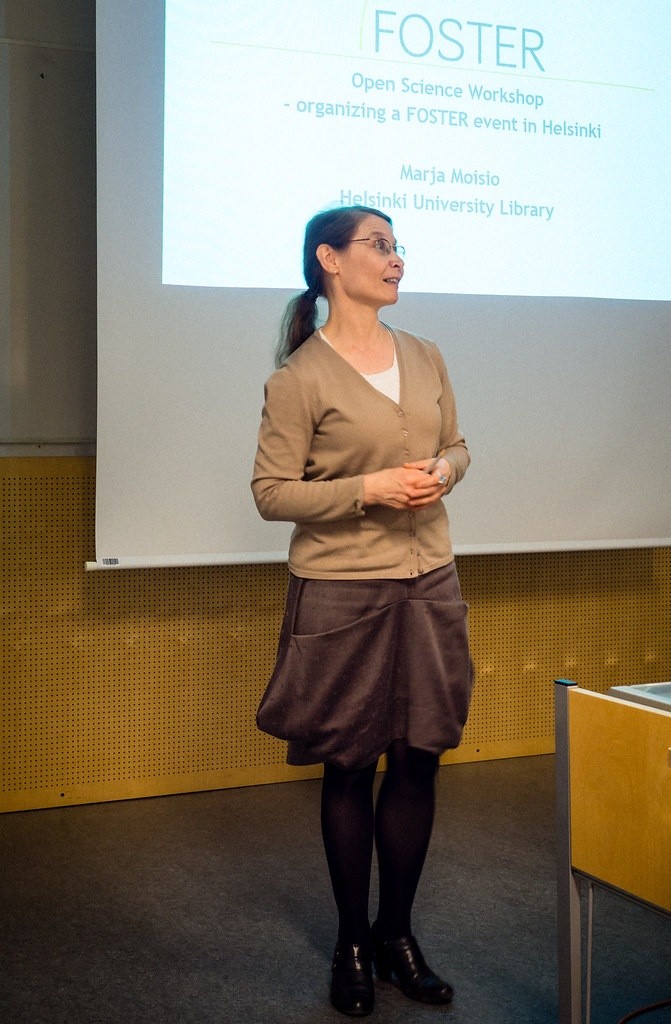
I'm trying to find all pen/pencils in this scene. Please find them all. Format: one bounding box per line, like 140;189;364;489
423;448;446;476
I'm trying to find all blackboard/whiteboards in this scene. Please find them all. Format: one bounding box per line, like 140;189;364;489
0;0;97;456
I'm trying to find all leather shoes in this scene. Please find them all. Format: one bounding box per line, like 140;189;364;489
331;922;454;1014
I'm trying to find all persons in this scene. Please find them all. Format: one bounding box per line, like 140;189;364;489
250;203;477;1017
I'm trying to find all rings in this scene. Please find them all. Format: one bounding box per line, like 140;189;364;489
438;475;455;490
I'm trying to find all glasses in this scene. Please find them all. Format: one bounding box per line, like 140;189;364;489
354;237;406;259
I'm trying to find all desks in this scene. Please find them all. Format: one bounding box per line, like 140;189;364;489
555;678;671;1024
